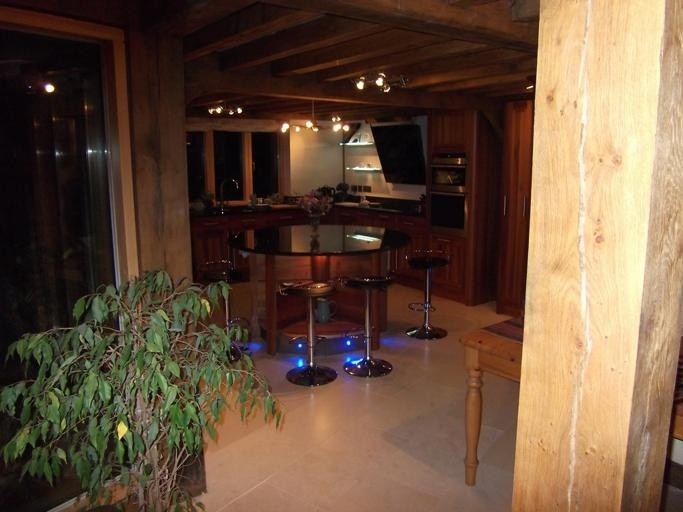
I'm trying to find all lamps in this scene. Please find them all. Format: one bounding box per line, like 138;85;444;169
280;100;350;137
355;72;408;94
207;103;244;116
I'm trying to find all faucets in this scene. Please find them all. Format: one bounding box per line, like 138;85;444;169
218;178;239;215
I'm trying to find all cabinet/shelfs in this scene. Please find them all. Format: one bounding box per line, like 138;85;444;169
428;231;465;290
395;216;428;282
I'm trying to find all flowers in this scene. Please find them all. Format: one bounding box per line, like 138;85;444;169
296;189;334;216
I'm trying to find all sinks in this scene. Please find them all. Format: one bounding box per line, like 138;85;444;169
204;205;272;213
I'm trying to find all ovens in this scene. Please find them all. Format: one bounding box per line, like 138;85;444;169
429;152;466;236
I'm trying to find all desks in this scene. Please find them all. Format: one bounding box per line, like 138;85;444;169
226;222;410;356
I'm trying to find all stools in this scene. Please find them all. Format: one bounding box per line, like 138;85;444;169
200;254;451;388
